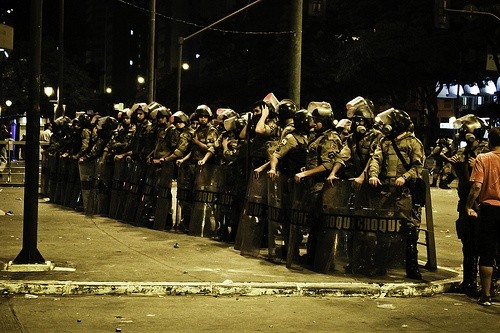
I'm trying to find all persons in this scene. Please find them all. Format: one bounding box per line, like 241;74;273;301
427;132;460;189
431;114;500;302
466;126;500;307
40;95;425;280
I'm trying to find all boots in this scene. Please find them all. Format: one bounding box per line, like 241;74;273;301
175;191;358;275
41;176;174;232
404;231;422;280
429;178;437;187
449;249;477;294
479;263;500;296
361;231;389;278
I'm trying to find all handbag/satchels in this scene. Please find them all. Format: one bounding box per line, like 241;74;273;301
407;178;426;208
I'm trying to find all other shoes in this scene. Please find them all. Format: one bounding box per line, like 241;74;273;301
477;296;492;306
440;180;451;189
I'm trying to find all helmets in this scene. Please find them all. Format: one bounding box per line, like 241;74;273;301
53;102;274;133
308;107;334;128
351;109;373;126
293;109;312;131
276;99;298;121
436;139;443;145
386;109;414;134
363;99;375;115
459;116;486;141
